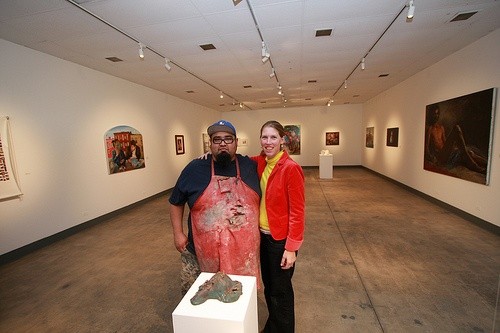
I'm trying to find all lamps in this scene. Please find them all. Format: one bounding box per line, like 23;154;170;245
360;58;366;70
404;0;415;19
327;96;334;107
344;80;347;89
163;58;172;71
260;41;288;110
137;42;146;58
218;90;250;111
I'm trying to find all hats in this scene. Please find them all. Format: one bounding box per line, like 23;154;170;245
207;120;236;138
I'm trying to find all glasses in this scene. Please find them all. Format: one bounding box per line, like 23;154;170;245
212;137;235;144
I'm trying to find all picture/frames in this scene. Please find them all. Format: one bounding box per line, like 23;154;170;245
174;135;186;155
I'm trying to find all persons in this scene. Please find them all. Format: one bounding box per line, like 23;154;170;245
169;119;263;292
198;121;306;333
112;141;126;168
124;140;140;163
